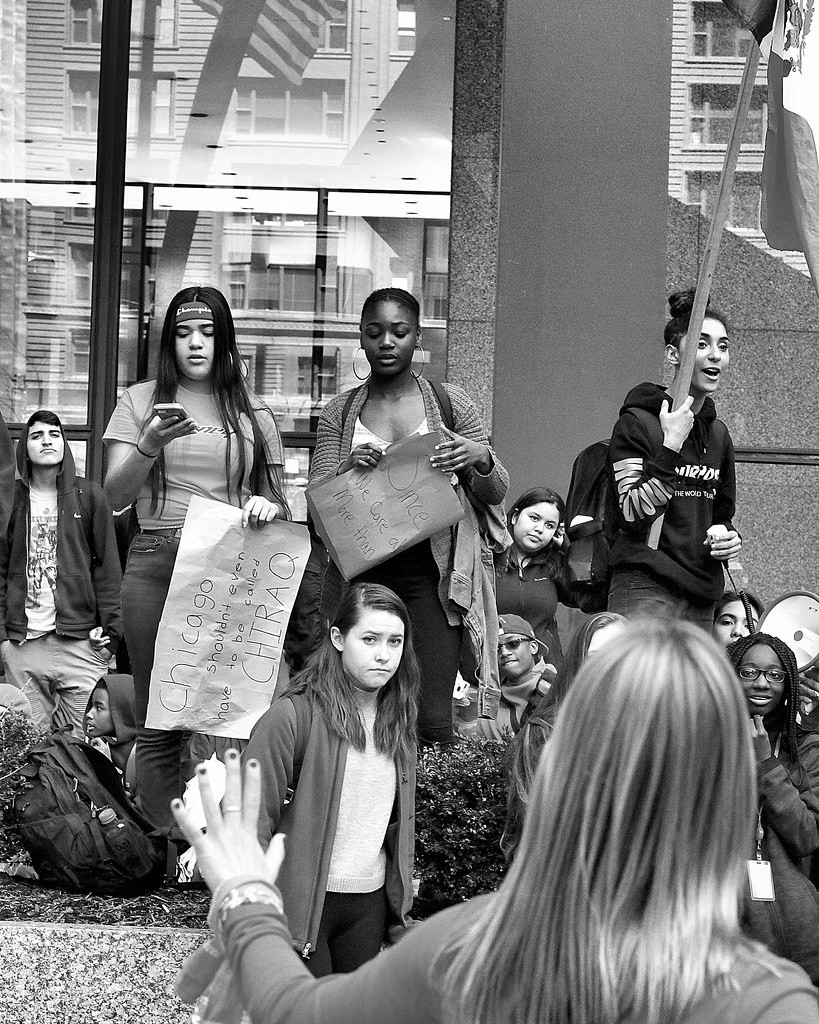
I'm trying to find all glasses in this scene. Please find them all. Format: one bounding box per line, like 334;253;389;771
497;638;533;655
737;666;787;683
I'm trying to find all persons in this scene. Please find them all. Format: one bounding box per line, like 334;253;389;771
0;288;819;1024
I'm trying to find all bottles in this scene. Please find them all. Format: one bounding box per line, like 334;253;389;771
99;809;153;879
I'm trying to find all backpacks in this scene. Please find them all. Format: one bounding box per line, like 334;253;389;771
550;407;725;614
16;724;168;899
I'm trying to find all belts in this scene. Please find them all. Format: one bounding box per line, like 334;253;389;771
143;527;182;538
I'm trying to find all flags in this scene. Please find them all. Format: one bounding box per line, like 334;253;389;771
192;0;347;88
722;0;819;297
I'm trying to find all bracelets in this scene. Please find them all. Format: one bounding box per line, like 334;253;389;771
136;444;158;458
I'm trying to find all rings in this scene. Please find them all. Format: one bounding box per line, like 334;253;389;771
221;806;242;814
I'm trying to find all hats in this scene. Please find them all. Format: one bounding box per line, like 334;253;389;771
497;614;549;656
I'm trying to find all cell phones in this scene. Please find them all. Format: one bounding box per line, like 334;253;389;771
154;403;199;432
